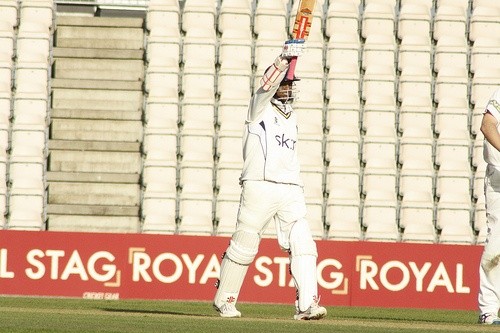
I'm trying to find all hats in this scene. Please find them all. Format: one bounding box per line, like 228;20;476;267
263;63;301;105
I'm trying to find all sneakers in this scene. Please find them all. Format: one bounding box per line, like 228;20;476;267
211;299;328;321
476;312;500;326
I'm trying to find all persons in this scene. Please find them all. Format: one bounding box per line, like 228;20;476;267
477;87;500;325
214;39;328;320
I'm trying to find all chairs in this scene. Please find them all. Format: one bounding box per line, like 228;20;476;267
0;0;499;246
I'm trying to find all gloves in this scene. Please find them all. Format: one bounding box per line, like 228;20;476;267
281;39;306;60
